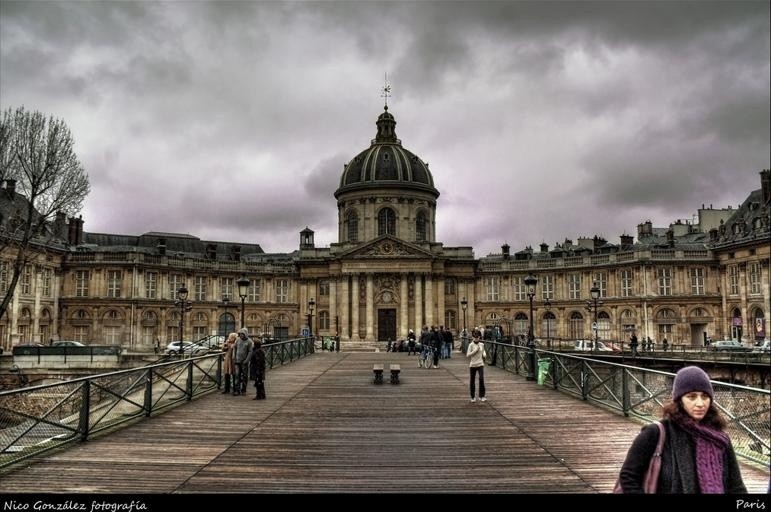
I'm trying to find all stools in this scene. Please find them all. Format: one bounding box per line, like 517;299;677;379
390;364;400;383
373;363;383;383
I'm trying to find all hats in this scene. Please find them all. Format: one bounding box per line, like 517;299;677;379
670;364;714;400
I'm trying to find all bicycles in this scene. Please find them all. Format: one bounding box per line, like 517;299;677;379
417;343;437;369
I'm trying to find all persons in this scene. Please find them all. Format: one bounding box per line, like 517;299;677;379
526;327;532;346
387;325;492;368
232;328;254;396
663;338;668;351
647;336;651;353
466;331;487;403
620;366;748;494
641;338;646;352
630;333;638;356
222;333;237;394
250;340;265;400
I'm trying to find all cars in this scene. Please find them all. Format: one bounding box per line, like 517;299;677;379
760;339;770;351
473;325;505;338
12;342;48;354
46;340;88;355
386;340;438;354
709;341;754;356
0;346;4;354
198;334;280;351
576;339;630;353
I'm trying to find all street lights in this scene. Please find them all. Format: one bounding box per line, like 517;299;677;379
236;273;250;329
223;295;230;352
460;296;468;353
525;271;539;380
586;279;605;355
544;297;552;350
175;282;193;354
309;298;316;352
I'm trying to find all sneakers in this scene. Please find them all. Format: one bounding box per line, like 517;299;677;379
470;398;476;403
479;397;486;402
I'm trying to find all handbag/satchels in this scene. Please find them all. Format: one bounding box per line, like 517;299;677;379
613;421;666;494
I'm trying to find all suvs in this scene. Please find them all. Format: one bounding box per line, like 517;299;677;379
164;341;209;354
